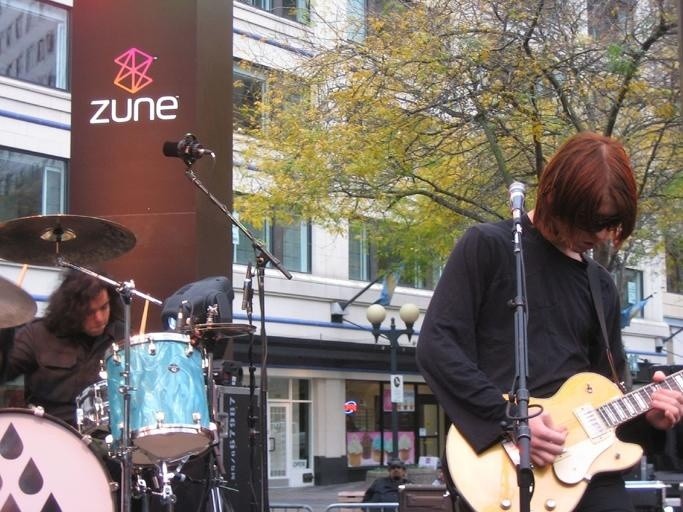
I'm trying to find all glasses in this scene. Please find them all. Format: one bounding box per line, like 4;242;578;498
561;209;621;232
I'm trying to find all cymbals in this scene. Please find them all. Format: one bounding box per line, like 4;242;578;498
0;276;37;330
0;214;137;267
179;323;256;342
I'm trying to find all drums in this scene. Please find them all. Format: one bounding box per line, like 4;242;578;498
96;332;217;468
76;381;115;463
0;405;120;512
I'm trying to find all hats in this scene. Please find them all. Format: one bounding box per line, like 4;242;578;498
388;461;405;470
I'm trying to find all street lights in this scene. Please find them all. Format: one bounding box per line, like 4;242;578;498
366;303;421;461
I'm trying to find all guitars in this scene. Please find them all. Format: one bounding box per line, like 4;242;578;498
446;371;683;512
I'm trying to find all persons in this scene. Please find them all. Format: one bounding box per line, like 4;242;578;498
1;265;209;512
361;458;416;512
432;462;446;485
415;131;683;512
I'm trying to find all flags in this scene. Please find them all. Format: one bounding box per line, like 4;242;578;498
377;265;405;306
620;301;648;331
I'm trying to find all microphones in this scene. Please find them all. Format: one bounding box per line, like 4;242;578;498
163;141;212;157
509;182;526;253
241;267;253;311
